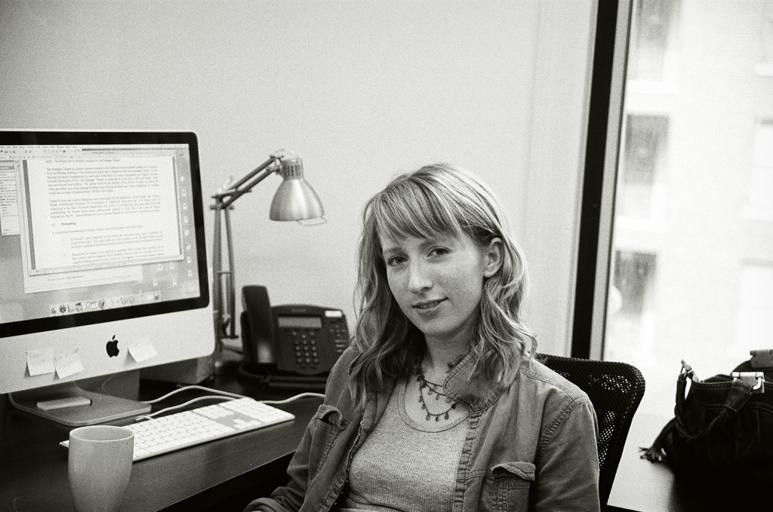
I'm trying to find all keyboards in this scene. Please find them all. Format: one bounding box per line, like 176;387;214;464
59;396;296;462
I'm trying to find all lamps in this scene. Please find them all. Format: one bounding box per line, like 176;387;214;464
209;149;328;360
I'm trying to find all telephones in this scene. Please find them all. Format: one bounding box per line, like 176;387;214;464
236;284;352;392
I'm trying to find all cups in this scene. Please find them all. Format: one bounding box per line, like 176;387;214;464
66;425;134;512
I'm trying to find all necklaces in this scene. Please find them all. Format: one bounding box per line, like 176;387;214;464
412;351;465;425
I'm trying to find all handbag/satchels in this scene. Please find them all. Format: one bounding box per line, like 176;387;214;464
639;361;772;476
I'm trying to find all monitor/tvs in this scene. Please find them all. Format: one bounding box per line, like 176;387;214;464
0;128;217;426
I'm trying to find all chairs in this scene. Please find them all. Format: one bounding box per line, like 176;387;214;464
534;354;645;512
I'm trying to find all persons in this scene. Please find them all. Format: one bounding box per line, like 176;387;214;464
243;161;601;511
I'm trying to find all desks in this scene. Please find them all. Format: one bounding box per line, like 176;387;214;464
1;348;325;512
